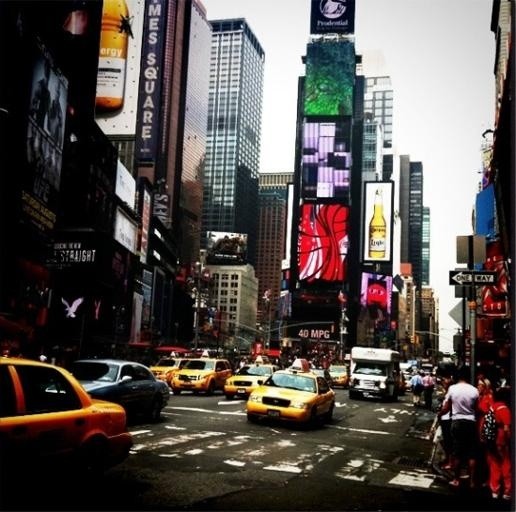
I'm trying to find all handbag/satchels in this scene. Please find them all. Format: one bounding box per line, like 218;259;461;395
415;384;424;392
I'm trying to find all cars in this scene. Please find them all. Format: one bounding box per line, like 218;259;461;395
0;360;136;475
45;357;171;426
326;363;347;388
170;360;233;396
245;358;338;429
224;364;281;398
148;358;192;386
400;358;438;392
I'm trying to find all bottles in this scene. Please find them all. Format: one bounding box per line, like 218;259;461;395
92;1;130;117
367;188;387;259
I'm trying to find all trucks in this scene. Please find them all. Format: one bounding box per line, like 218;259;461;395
348;345;402;401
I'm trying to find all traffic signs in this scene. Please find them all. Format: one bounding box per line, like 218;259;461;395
449;270;497;286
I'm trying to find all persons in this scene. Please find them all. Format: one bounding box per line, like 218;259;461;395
438;363;515;500
421;371;435;409
409;369;423;406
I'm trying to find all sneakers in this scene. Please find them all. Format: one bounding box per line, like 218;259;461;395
441;460;512;500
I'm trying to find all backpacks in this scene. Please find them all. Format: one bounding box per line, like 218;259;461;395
481;405;508;442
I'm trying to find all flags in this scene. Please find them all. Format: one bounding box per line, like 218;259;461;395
476;184;499;240
